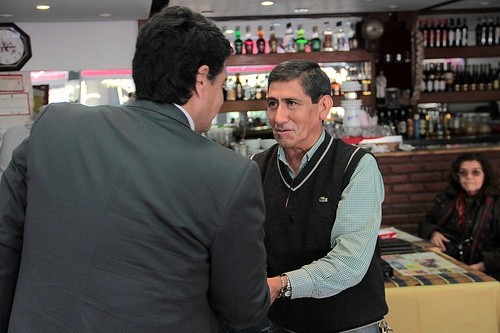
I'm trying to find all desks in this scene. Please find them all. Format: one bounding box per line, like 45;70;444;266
378;225;500;333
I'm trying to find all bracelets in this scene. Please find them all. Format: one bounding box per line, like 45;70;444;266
277;275;283;298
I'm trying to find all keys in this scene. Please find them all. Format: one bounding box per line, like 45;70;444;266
378;320;394;333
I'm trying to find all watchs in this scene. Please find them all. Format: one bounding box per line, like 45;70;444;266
283;273;292;299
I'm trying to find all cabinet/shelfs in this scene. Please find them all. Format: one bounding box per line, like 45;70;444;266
419;46;500;103
220;50;376;115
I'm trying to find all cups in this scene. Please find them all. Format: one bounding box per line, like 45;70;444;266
208;124;233;148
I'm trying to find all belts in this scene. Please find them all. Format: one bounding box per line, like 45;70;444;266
346;320;389;333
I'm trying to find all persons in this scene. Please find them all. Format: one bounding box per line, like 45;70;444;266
417;151;500;281
248;57;389;333
0;5;271;333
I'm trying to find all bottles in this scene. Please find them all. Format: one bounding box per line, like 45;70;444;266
222;73;268;101
380;106;491;140
220;21;358;54
330;69;388;97
420;62;500;92
417;16;500;48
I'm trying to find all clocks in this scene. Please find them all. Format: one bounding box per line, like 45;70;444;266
0;22;32;72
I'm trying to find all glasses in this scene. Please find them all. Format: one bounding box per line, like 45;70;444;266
457;170;481;177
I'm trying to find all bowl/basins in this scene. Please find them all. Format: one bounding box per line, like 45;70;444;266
243;139;277;154
